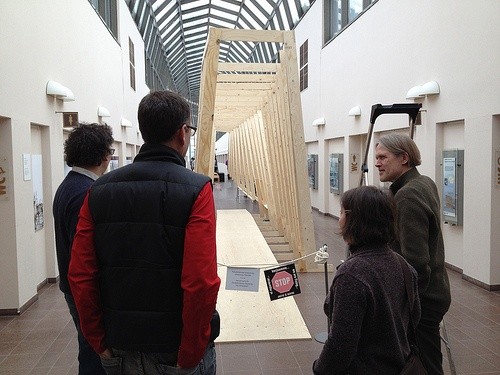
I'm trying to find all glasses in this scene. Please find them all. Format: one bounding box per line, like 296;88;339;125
107;149;115;155
186;126;197;136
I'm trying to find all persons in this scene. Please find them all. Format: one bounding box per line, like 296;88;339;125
51;123;114;375
313;185;421;375
67;91;220;375
191;157;195;171
375;133;451;375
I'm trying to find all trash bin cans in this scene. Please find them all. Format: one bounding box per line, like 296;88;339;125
219;173;224;182
